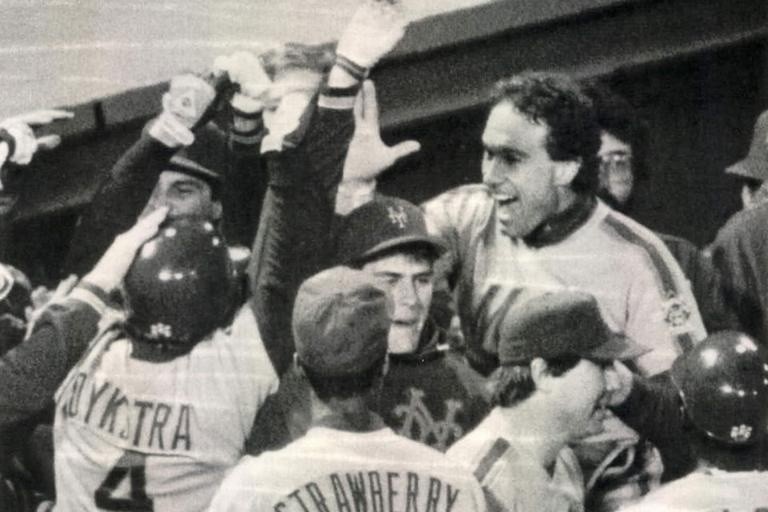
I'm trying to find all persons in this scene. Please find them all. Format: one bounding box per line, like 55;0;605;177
0;0;767;512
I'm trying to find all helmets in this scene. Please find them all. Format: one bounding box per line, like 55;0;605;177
123;217;242;344
671;332;768;445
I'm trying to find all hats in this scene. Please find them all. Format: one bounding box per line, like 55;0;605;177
724;111;768;183
498;292;653;364
292;264;391;377
331;194;446;265
140;115;228;185
